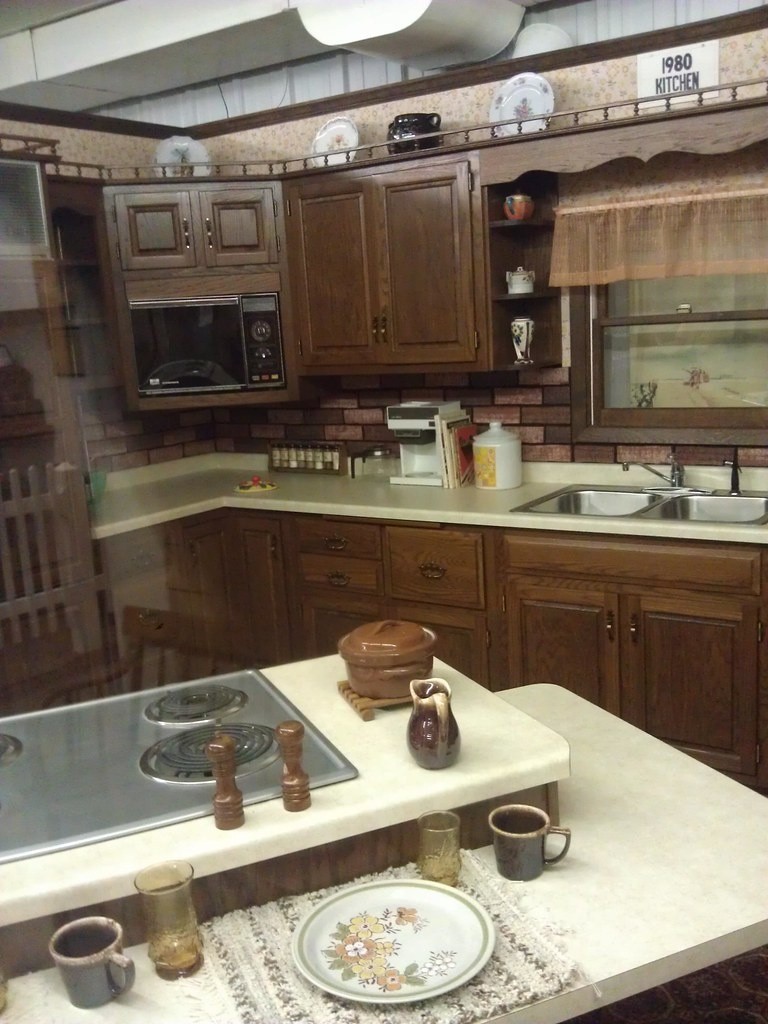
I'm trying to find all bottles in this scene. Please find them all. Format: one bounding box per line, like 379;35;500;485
271;444;339;471
471;421;522;489
511;317;534;365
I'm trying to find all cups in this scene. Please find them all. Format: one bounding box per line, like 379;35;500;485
417;811;462;888
408;677;462;771
49;915;136;1008
506;266;536;295
489;804;573;881
503;188;534;221
133;860;204;980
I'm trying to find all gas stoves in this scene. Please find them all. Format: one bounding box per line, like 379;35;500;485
1;667;360;865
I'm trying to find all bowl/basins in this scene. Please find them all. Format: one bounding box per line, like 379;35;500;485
336;618;438;698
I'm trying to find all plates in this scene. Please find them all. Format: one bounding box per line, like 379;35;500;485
291;878;497;1004
488;72;556;138
153;136;211;178
310;118;358;168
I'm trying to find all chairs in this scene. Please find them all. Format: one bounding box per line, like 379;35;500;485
41;605;257;710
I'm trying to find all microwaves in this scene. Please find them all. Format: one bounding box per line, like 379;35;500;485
127;292;286;398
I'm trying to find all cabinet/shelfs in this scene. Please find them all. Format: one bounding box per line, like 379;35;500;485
46;181;121;392
282;151;491;378
279;512;493;693
101;180;288;282
486;171;562;371
493;526;768;797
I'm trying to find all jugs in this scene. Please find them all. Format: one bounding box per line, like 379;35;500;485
350;444;396;486
386;113;441;154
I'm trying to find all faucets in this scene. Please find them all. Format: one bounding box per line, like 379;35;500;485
623;458;684;492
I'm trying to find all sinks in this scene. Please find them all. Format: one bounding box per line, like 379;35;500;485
642;490;768;526
508;483;662;521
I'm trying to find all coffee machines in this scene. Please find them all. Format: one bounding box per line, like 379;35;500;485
387;399;466;487
